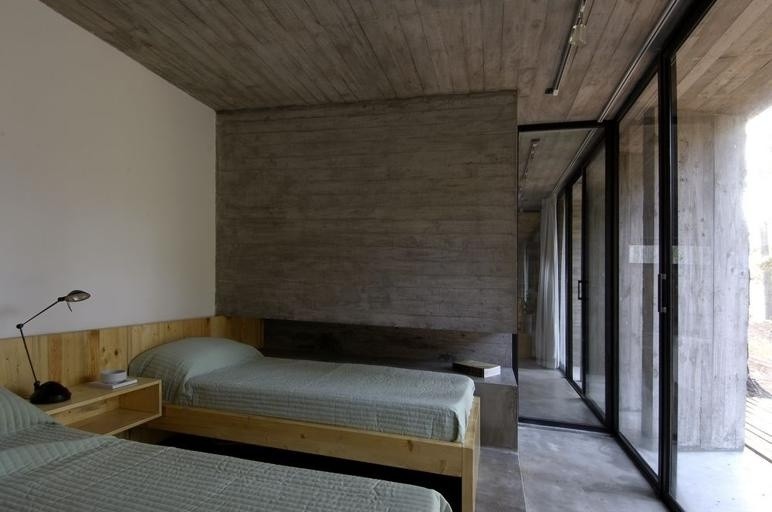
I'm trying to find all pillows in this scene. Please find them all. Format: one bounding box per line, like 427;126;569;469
0;388;63;430
128;336;265;382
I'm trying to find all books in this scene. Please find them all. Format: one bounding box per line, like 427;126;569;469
453;360;501;379
87;378;138;390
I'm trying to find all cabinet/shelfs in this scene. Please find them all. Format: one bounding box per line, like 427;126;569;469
30;376;162;437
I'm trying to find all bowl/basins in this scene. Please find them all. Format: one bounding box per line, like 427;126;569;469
100;369;127;384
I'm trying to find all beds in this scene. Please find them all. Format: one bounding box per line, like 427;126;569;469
127;337;481;511
1;388;454;512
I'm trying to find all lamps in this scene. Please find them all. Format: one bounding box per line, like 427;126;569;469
16;290;91;404
